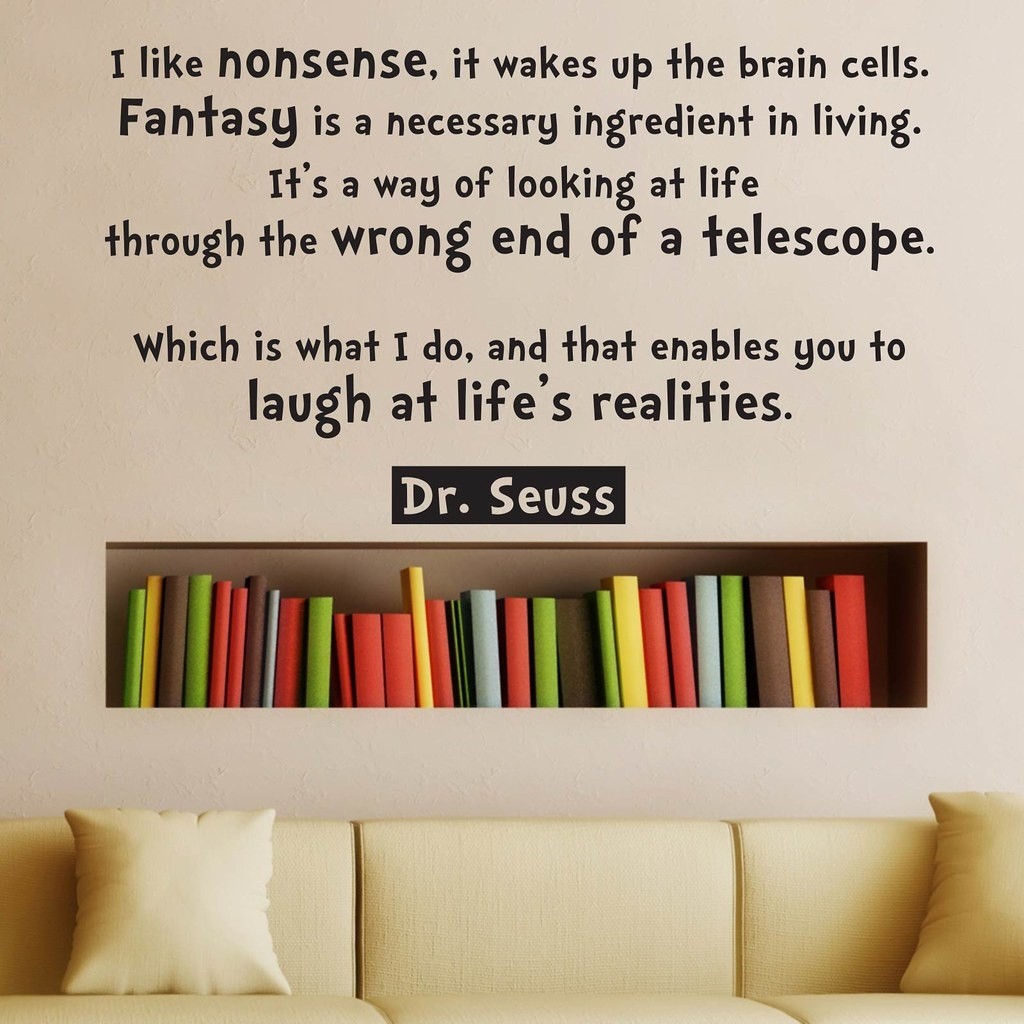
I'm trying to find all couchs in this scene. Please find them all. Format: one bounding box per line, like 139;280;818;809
0;811;1024;1024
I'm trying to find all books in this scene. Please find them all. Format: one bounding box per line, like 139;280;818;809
124;565;869;707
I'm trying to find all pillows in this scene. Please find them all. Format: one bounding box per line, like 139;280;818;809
898;794;1023;991
59;807;293;997
